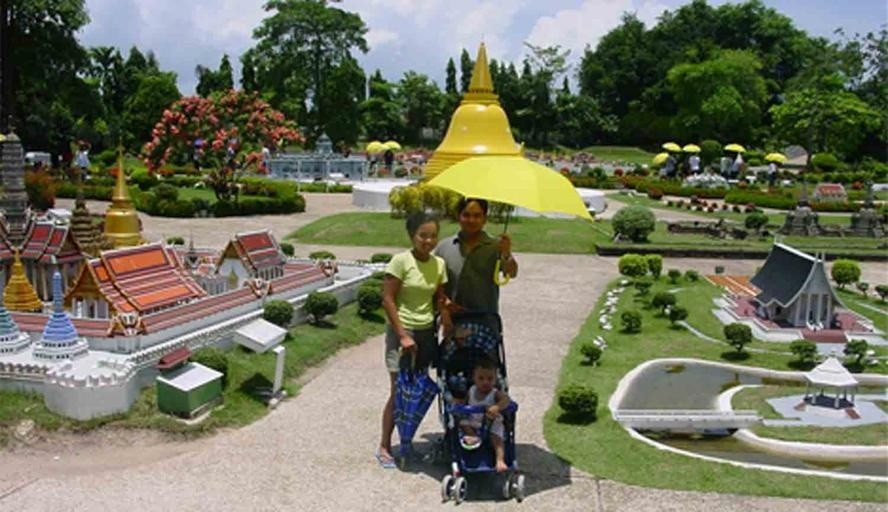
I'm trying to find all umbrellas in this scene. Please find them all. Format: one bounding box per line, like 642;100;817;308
764;152;788;162
653;152;669;164
683;143;701;152
424;142;594;287
723;143;747;153
661;141;681;152
366;140;383;153
388;345;443;469
384;139;401;151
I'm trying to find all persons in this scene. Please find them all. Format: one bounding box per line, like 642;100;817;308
736;157;749;179
369;155;377;169
767;161;778;191
383;150;394;172
458;356;511;475
684;154;689;176
688;152;701;173
721;153;733;179
375;211;454;470
666;152;677;176
658;163;668;176
434;197;519;313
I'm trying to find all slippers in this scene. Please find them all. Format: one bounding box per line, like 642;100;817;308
375;448;397;468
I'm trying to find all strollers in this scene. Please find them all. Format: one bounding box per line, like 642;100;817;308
432;309;527;506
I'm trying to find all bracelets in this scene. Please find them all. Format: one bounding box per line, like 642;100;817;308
500;252;513;262
